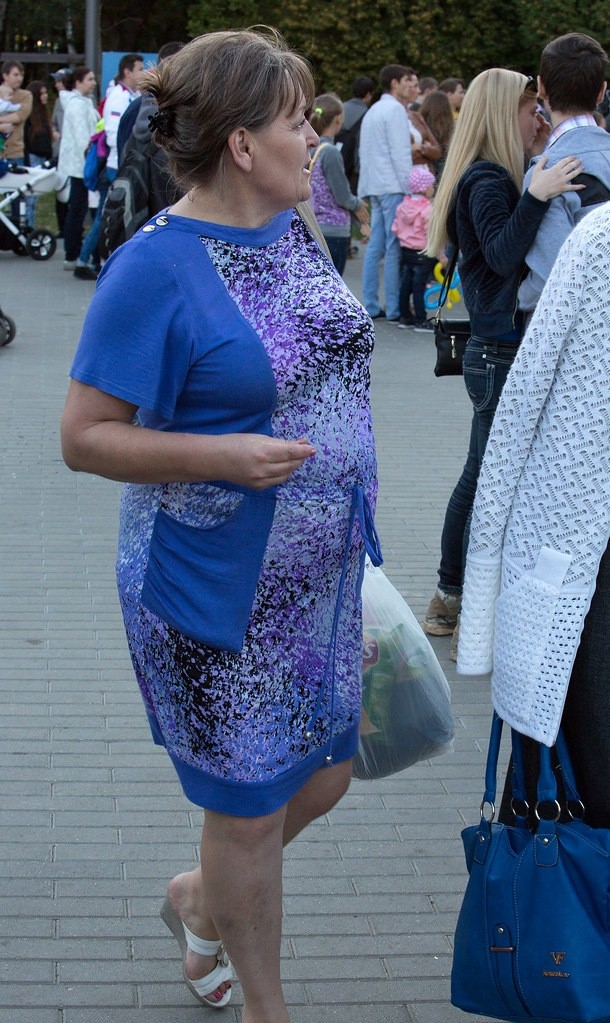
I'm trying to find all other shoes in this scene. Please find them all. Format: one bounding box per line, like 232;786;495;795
387;316;399;325
424;587;461;636
371;310;386;320
63;260;76;269
73;266;98;280
451;613;462;662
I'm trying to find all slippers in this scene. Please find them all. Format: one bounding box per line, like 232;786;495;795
160;899;232;1007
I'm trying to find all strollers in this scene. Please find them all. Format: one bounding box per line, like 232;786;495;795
0;155;59;262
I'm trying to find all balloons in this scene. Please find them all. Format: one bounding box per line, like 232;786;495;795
425;262;463;309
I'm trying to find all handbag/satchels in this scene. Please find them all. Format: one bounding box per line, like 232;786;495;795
83;142;98;191
451;708;610;1023
350;557;454;780
434;316;471;377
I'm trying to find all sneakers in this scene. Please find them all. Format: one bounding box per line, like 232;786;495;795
398;317;415;328
412;321;434;332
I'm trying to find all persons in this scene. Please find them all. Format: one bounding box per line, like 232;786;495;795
336;76;374;259
73;54;145;279
405;67;442;167
0;60;53;229
391;169;448;332
56;65;101;270
411;77;467;173
359;65;413;326
308;95;372;278
426;31;610;834
117;42;182;219
49;68;71;239
61;30;376;1023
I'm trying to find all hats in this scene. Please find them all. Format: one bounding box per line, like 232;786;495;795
409;166;434;194
50;68;72;80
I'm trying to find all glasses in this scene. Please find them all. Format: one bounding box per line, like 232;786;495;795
524;75;538;94
